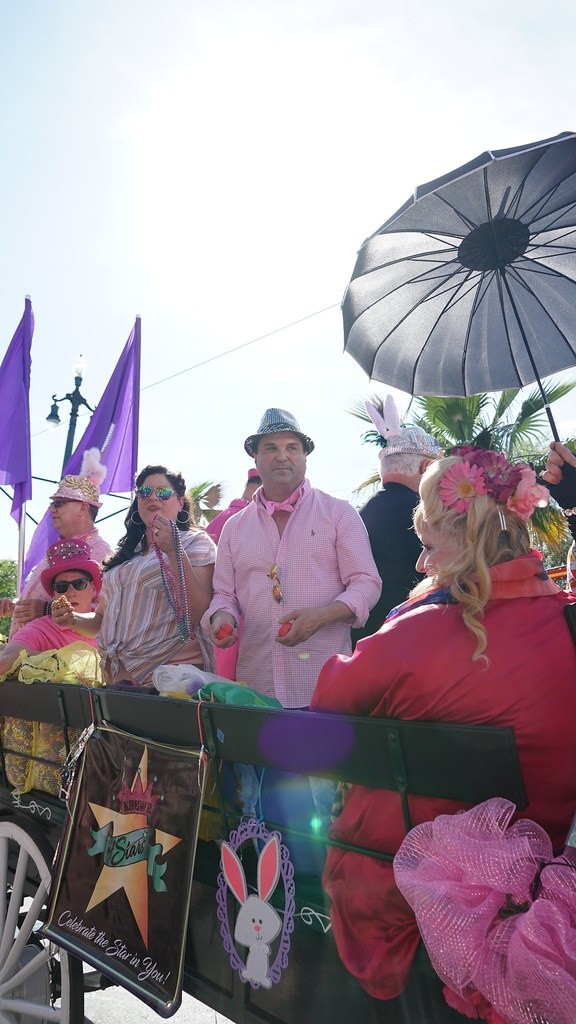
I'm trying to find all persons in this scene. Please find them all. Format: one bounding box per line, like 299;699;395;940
1;498;116;643
306;435;576;1001
542;441;576;543
200;408;382;839
350;426;440;652
566;538;576;593
50;464;217;692
204;467;262;679
0;539;103;677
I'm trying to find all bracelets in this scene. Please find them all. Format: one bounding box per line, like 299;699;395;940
44;600;51;616
562;505;576;517
72;611;77;625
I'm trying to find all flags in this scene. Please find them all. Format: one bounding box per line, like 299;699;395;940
0;298;35;525
60;316;141;493
19;502;63;594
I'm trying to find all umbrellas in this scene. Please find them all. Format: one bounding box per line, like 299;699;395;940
342;130;576;443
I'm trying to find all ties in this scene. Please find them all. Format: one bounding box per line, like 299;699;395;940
267;563;284;604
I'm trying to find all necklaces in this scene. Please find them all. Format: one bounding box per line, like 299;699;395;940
150;514;196;643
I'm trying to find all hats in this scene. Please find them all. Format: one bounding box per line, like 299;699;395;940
365;394;444;460
49;447;107;507
40;538;103;600
246;469;261;479
243;408;315;458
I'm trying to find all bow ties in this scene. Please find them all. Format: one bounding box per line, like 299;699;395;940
260;477;306;516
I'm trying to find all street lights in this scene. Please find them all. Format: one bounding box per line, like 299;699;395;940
44;351;98;486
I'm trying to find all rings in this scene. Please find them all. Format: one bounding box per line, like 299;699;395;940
155;529;158;534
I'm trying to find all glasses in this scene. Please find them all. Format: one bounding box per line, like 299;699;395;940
134;485;181;502
50;499;81;508
51;578;93;594
